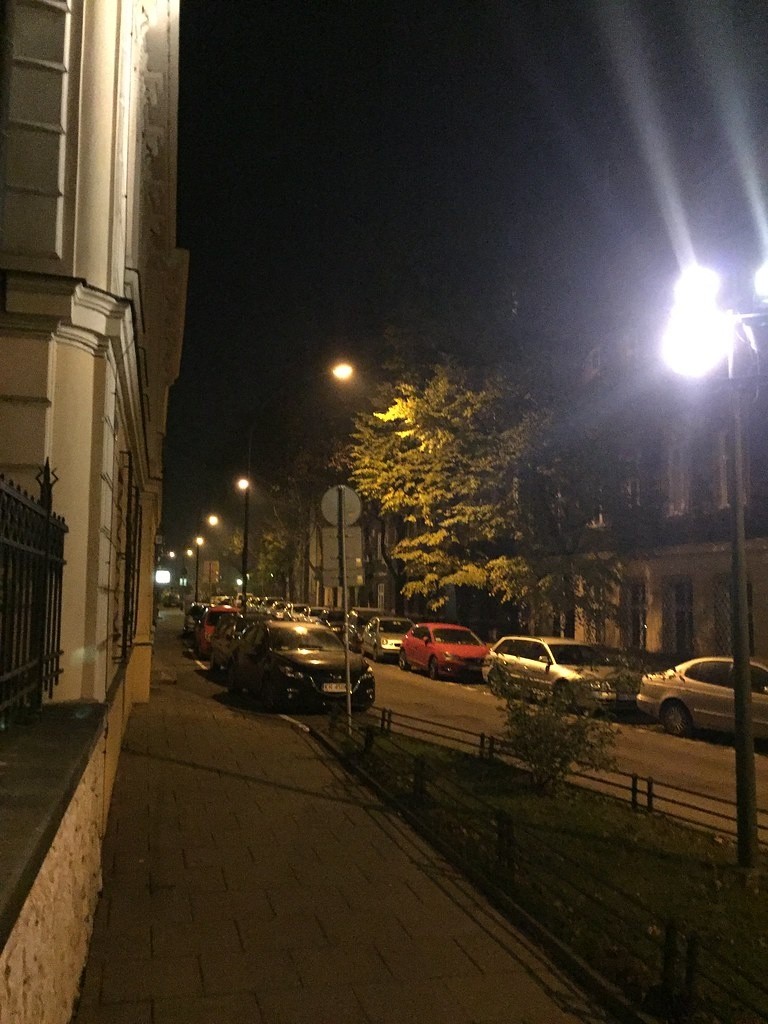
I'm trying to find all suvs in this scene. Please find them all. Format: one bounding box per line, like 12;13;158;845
481;636;642;717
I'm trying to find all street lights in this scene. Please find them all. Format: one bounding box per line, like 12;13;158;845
195;508;219;602
182;545;193;586
237;478;249;608
659;258;768;862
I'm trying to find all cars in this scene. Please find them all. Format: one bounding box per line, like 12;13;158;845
360;616;415;663
184;592;385;660
635;658;768;741
398;623;491;681
226;621;375;712
